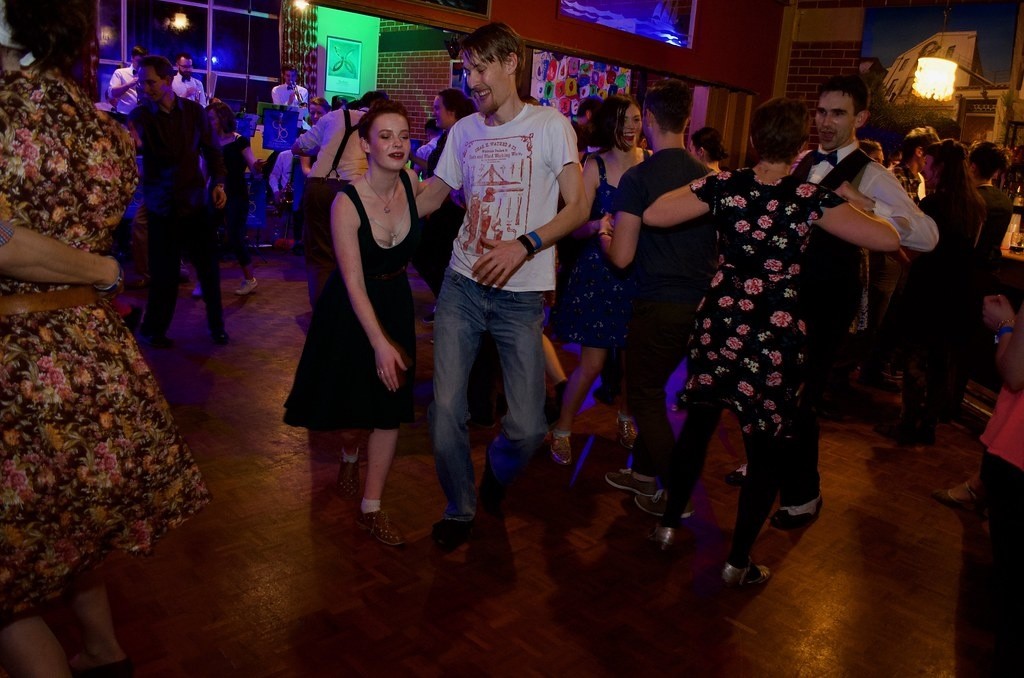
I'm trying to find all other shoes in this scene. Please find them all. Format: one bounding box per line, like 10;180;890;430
549;323;997;507
604;468;660;497
479;446;507;511
634;489;696;519
616;412;638;449
431;518;472;551
549;431;572;466
338;456;360;497
721;556;770;586
358;506;405;545
69;656;134;678
769;490;822;530
725;463;747;485
109;234;307;346
647;525;673;555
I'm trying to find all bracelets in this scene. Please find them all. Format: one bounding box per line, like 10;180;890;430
529;231;542;249
93;255;122;293
517;235;534;256
997;326;1013;337
996;319;1015;331
599;232;612;236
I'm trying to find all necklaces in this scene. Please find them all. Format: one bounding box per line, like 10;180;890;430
364;174;398;214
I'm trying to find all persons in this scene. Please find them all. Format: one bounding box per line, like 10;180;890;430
0;0;213;678
108;46;266;350
980;292;1024;678
272;22;1013;590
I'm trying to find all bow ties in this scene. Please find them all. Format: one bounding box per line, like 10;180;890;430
133;70;139;75
182;76;190;82
287;86;293;90
810;150;838;167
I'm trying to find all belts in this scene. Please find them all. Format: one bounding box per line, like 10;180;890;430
364;262;410;280
0;285;100;316
304;177;350;185
